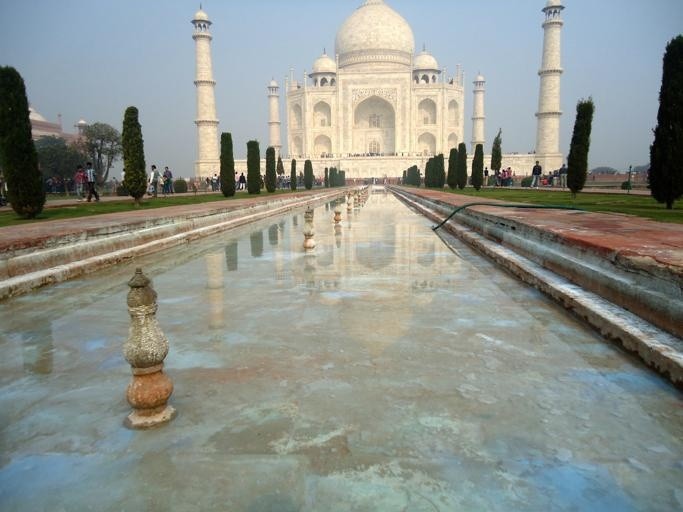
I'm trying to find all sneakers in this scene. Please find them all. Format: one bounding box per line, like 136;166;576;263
148;191;174;197
76;195;99;202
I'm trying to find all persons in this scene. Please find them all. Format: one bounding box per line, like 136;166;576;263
120;168;125;186
43;176;73;194
496;166;512;186
83;162;101;202
234;171;239;190
239;172;246;190
147;164;173;198
483;166;488;186
528;160;541;188
110;176;117;193
259;175;266;189
542;163;567;188
204;172;222;192
71;164;87;202
278;175;290;188
